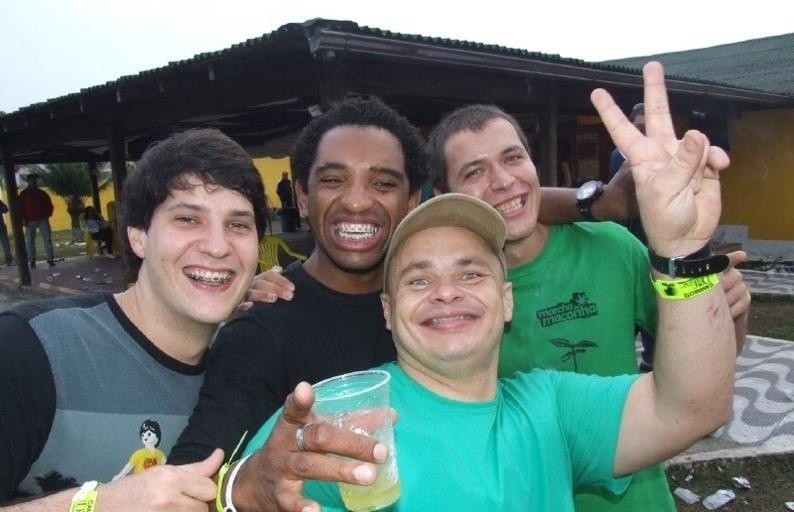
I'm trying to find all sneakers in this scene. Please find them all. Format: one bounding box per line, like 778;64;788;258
5;262;15;266
106;252;117;259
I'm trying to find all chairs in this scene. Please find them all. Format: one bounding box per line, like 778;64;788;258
258;237;307;274
78;212;107;261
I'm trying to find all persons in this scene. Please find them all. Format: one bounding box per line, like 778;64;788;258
275;170;291;207
607;102;656;372
424;106;752;511
207;60;737;511
165;98;639;512
17;174;57;269
1;199;15;267
2;130;268;512
82;206;114;260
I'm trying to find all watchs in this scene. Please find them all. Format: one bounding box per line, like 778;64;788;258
648;241;730;277
575;179;608;222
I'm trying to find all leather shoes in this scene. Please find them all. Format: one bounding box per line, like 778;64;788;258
46;260;55;265
30;262;36;268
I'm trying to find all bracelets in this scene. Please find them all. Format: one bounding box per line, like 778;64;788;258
70;480;102;512
217;459;248;512
226;452;252;511
648;274;725;301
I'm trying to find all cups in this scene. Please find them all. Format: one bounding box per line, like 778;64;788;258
303;371;402;512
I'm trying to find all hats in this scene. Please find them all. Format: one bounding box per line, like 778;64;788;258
383;192;508;291
282;171;288;176
